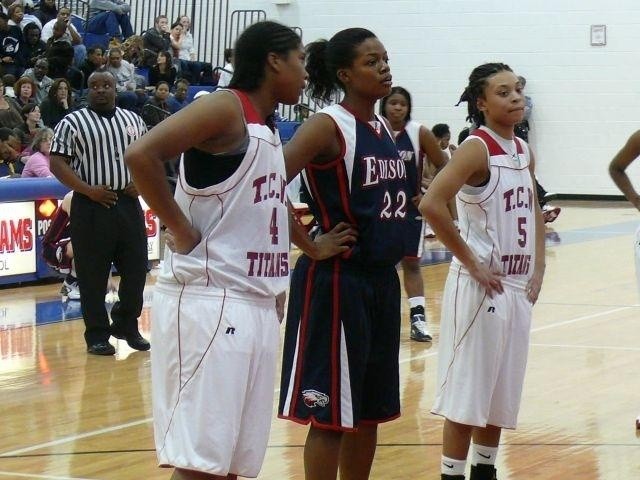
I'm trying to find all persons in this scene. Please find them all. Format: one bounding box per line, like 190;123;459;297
607;129;640;297
121;18;310;480
274;26;420;480
41;189;122;305
513;75;533;143
416;61;546;480
379;85;446;344
48;67;154;355
426;122;456;180
532;172;560;226
0;0;234;179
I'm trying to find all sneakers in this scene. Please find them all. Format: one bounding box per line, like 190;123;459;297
111;323;150;351
60;279;80;299
543;207;561;224
410;313;432;341
86;343;115;355
